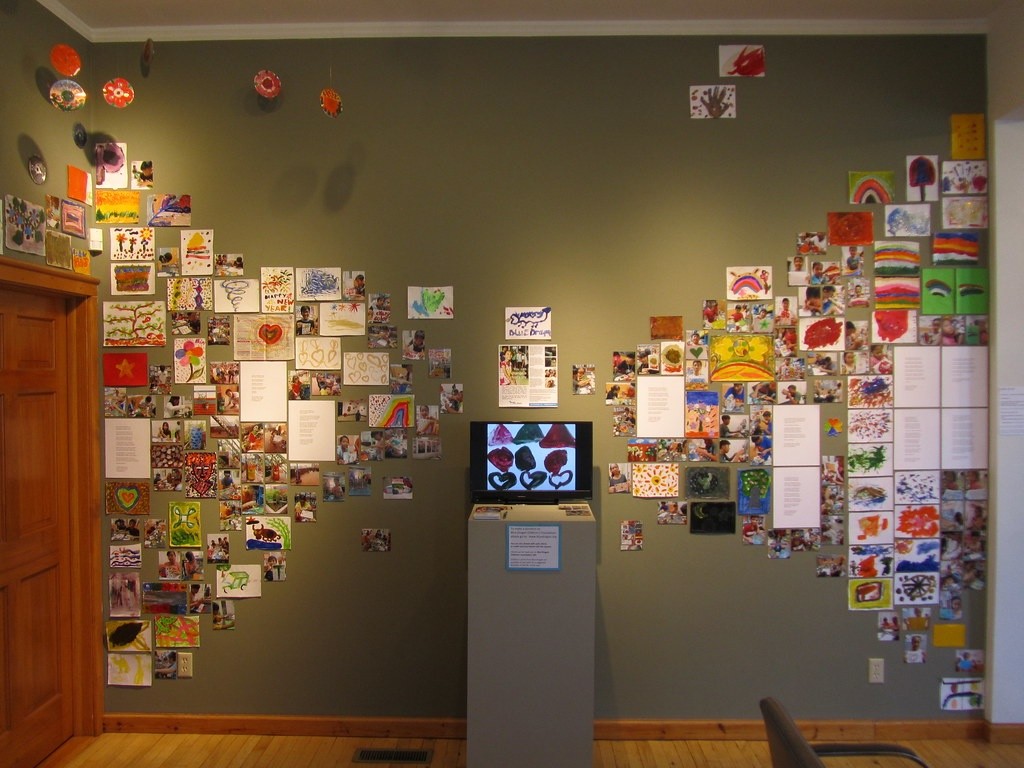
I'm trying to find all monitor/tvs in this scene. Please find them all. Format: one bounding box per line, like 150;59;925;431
469;420;593;505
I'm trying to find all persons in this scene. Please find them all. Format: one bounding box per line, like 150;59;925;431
957;652;976;672
939;469;987;622
880;617;901;640
105;250;463;581
500;347;557;389
904;607;931;630
155;651;176;678
604;232;845;575
903;636;927;663
190;583;204;611
138;161;153;188
843;246;989;374
574;366;590;391
212;602;235;630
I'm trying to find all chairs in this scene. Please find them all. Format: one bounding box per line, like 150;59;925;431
759;696;929;768
